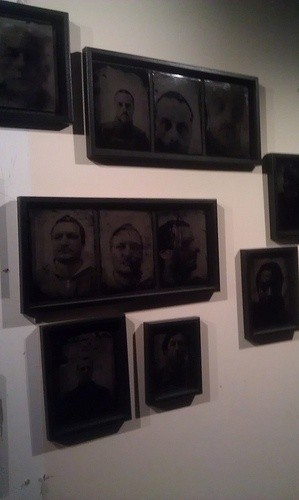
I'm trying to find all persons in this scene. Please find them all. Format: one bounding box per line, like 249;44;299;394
0;24;53;110
33;214;98;298
157;219;208;289
150;327;198;395
60;342;115;418
102;223;154;296
251;262;293;330
97;89;153;152
205;79;251;157
154;91;193;155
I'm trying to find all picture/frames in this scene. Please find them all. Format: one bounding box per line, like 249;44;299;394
0;0;299;446
